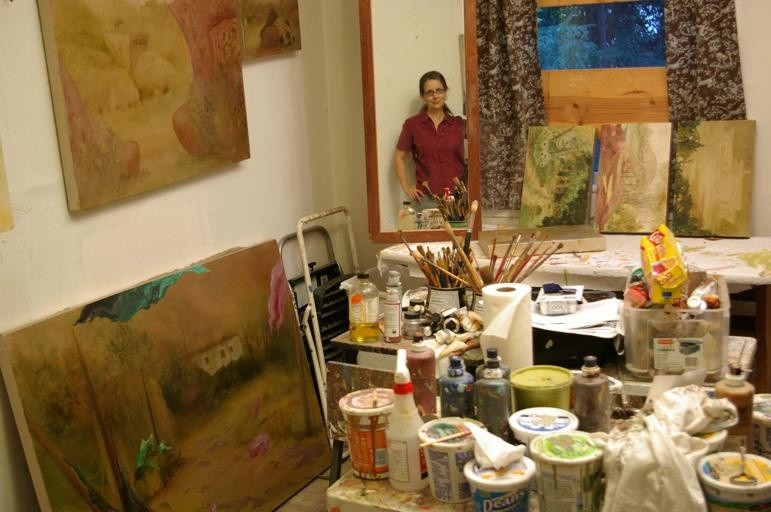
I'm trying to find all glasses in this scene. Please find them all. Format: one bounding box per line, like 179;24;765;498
423;88;445;97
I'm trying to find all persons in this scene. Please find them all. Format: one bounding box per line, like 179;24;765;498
394;71;467;209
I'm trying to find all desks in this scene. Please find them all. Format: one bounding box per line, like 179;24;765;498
325;470;481;511
378;210;771;390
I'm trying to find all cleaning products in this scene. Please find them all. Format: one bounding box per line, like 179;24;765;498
383;349;431;494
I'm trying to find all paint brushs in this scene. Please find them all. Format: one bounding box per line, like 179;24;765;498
419;424;494;448
399;199;566;291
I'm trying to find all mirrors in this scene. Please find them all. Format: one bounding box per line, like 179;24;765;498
359;2;481;241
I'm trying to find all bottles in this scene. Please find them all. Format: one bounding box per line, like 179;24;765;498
348;271;379;344
569;354;614;433
715;363;755;454
687;296;708;318
403;298;462;339
398;201;416;230
441;346;510;438
384;269;402;341
408;329;437;418
